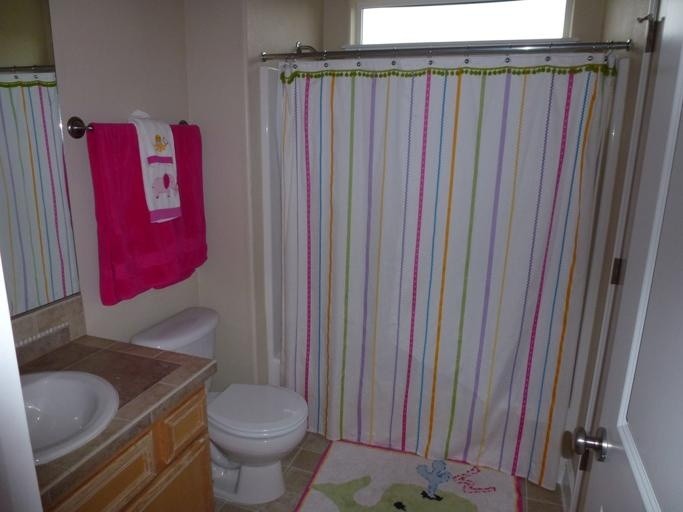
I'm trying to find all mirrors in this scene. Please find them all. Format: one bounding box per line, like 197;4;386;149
0;0;82;319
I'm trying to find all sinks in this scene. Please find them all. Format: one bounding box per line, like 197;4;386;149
20;371;120;465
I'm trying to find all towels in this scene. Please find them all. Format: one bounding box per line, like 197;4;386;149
127;117;183;223
87;123;208;305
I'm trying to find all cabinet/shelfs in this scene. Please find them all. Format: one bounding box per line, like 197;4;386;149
46;390;213;511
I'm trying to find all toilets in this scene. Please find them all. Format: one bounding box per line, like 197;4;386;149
132;306;308;506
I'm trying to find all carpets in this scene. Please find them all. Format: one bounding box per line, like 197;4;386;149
294;442;522;512
